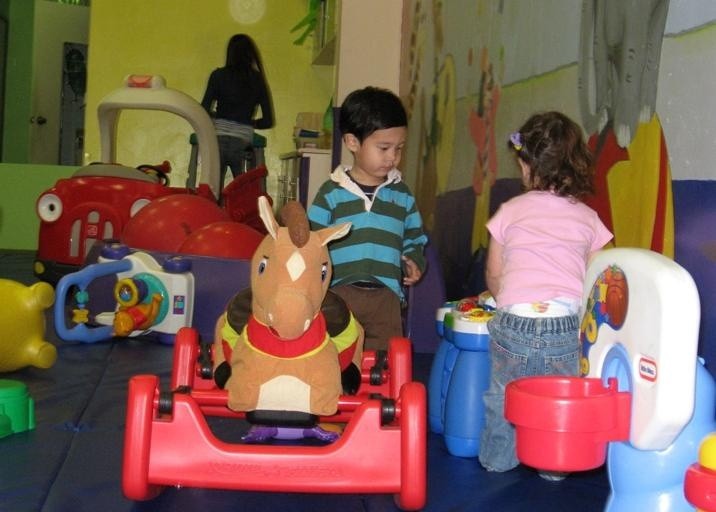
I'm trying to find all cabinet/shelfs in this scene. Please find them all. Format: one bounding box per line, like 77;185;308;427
275;148;332;227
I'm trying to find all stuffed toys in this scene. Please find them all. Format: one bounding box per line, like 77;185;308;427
213;194;368;414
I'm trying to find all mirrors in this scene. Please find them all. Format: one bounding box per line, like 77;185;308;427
2;2;98;172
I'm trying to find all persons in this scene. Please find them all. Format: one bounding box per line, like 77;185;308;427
199;33;277;187
307;84;427;365
479;111;616;480
